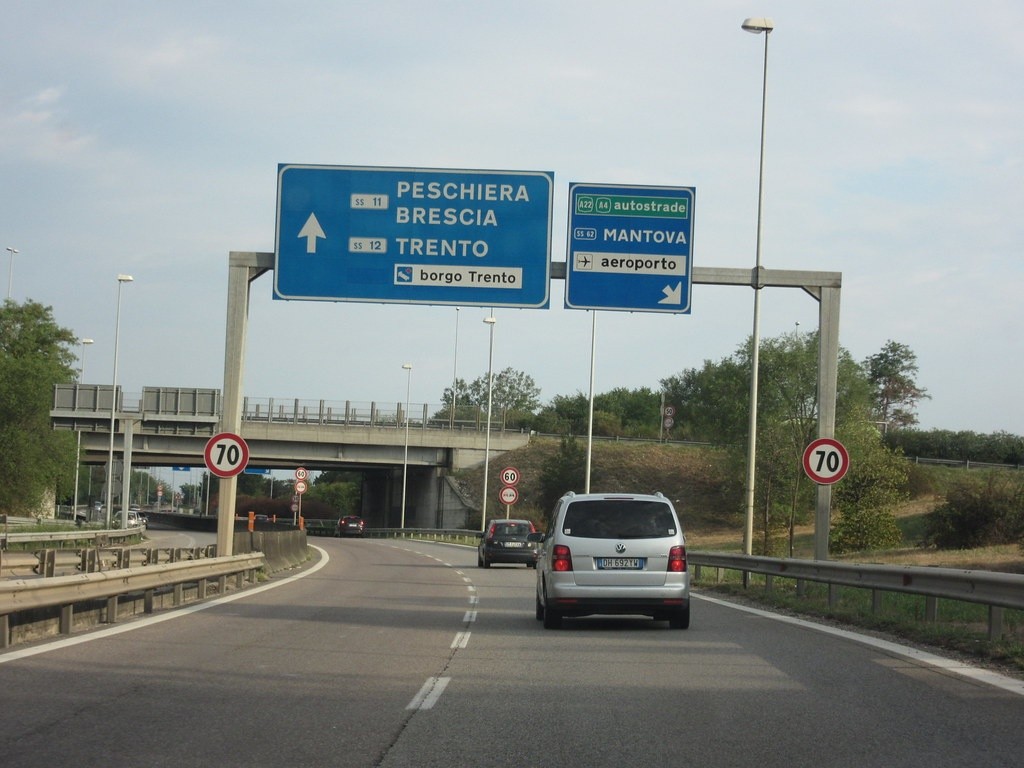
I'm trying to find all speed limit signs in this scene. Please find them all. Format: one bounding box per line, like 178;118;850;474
802;438;850;485
204;432;250;479
665;406;676;418
500;467;520;487
296;467;308;481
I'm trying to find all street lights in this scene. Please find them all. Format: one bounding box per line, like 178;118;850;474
452;306;460;408
80;338;94;383
740;16;775;581
5;246;20;300
103;272;134;529
481;317;496;533
402;363;413;529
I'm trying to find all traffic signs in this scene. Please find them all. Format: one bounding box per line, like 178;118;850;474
564;182;698;315
271;162;556;312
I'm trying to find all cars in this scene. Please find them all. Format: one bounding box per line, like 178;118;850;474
475;519;538;568
95;501;103;513
254;515;270;521
130;504;140;511
113;510;150;529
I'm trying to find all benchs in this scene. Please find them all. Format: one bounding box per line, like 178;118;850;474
496;529;527;534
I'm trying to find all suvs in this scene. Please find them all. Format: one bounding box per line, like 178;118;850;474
525;492;690;630
335;515;365;538
